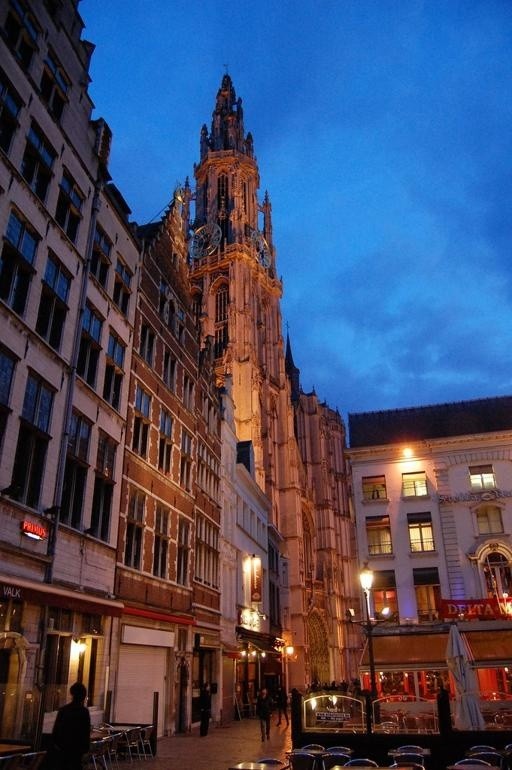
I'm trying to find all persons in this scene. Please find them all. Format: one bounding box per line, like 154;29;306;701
200;683;211;736
257;688;272;742
36;682;90;770
311;679;348;691
352;678;361;707
276;688;289;727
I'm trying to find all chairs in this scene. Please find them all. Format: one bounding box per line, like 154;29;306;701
0;722;155;769
255;742;512;769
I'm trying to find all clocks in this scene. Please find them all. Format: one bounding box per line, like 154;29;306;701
250;231;272;269
188;223;222;260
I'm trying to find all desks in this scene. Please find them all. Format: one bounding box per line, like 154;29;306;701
227;762;290;770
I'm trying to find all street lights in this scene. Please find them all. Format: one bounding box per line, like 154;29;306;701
342;563;393;724
286;647;294;696
